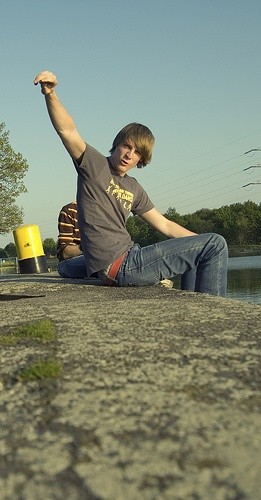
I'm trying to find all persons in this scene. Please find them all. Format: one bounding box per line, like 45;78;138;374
57;201;88;279
33;71;228;297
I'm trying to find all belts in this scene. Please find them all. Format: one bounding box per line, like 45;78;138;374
105;253;124;286
